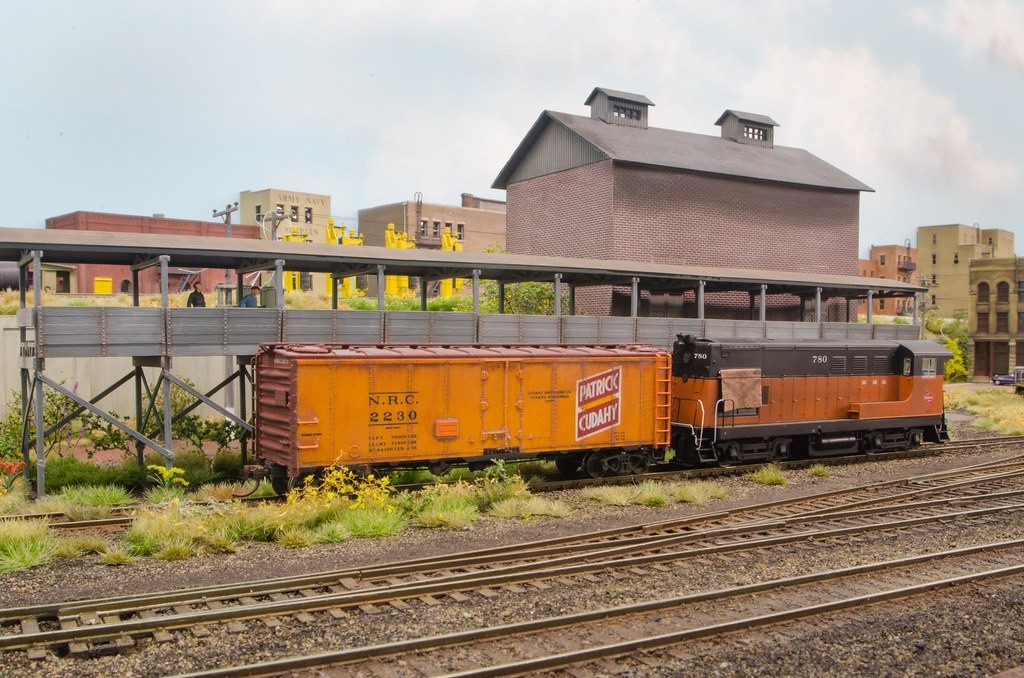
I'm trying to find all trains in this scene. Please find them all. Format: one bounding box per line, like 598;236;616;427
238;330;958;504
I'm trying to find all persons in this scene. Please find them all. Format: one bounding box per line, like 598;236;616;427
187;281;260;307
